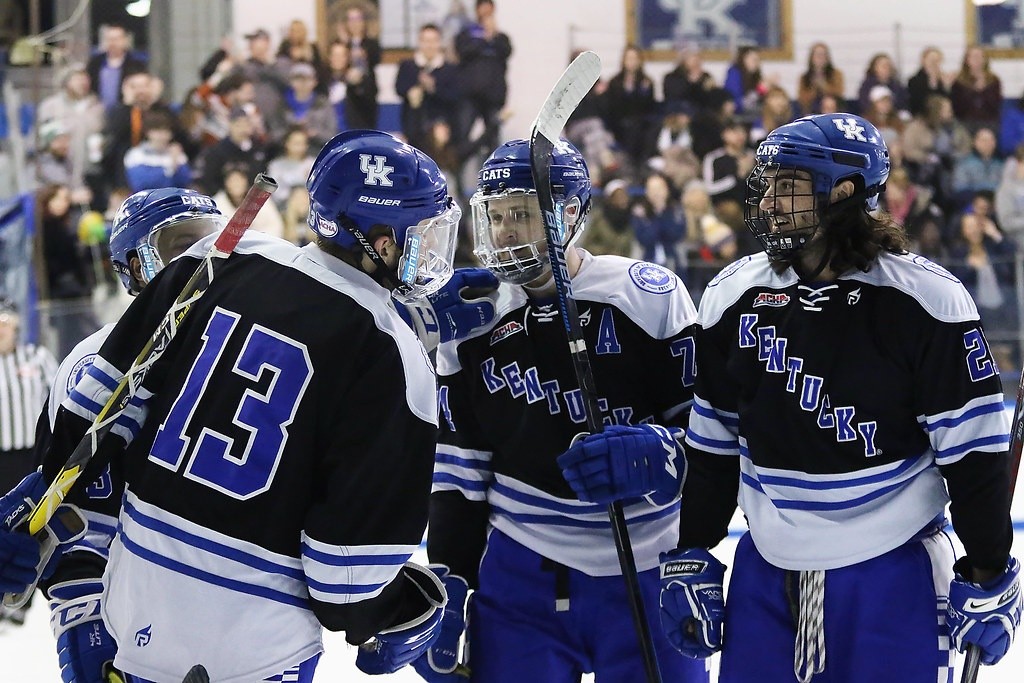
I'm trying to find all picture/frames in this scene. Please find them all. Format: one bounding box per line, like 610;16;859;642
314;0;479;65
624;0;796;63
965;0;1024;60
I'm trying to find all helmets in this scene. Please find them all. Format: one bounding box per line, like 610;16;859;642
475;137;590;284
109;188;222;293
744;113;892;274
305;130;461;304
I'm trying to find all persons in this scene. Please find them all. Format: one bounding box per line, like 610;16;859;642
34;186;232;683
563;41;1024;370
657;111;1024;683
0;0;513;622
426;134;711;682
0;128;500;683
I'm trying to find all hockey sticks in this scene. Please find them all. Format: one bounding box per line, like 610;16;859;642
532;50;663;683
18;172;278;537
961;367;1024;683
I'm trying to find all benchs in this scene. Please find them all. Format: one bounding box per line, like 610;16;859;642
615;99;1024;376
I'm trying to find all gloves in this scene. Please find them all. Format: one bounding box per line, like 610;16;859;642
559;424;688;507
410;576;469;682
0;473;89;597
45;579;122;683
657;548;727;654
357;563;450;673
946;555;1023;663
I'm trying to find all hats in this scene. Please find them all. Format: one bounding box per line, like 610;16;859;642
664;99;693;117
870;85;895;101
